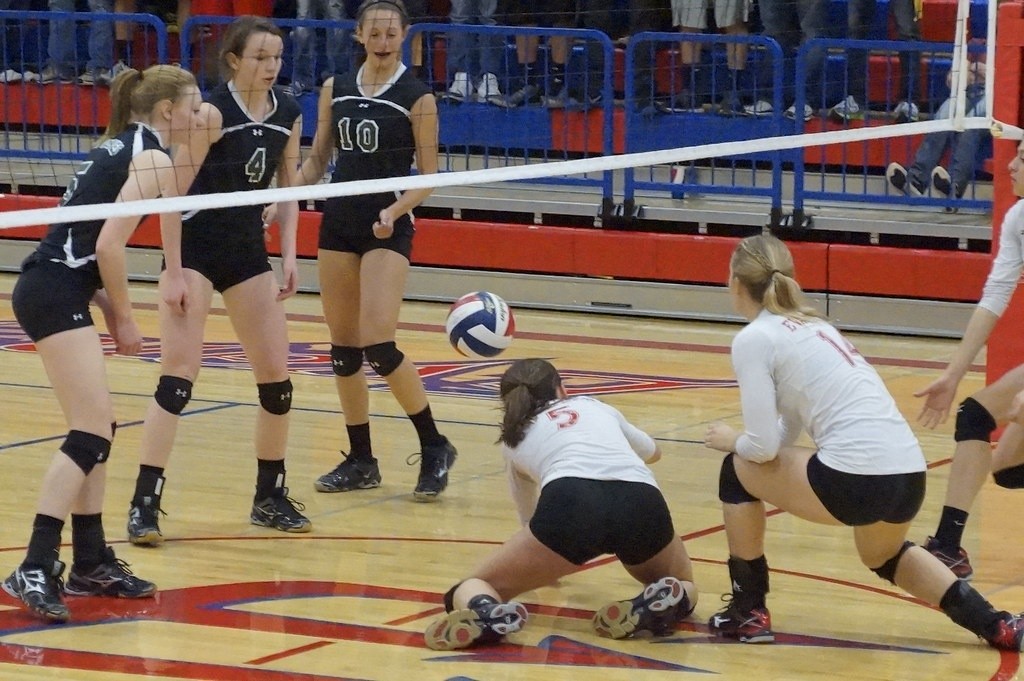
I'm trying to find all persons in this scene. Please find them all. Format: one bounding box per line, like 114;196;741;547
883;37;990;212
280;0;932;123
911;130;1024;578
127;15;313;543
0;63;207;622
702;234;1024;654
425;357;700;653
260;0;458;499
0;0;270;85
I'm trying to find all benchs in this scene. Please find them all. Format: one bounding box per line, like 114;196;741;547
0;0;992;178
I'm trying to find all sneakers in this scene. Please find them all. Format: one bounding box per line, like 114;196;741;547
921;536;974;581
895;100;918;123
593;576;687;640
828;95;865;120
407;435;459;499
985;610;1024;653
282;82;314;96
786;102;818;121
314;450;381;493
252;485;312;532
126;501;168;543
932;167;959;213
708;593;775;643
745;99;773;116
886;162;925;196
433;75;742;118
1;58;132;89
423;601;527;650
64;545;157;599
2;559;71;622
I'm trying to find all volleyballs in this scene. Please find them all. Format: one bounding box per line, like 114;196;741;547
445;289;514;359
